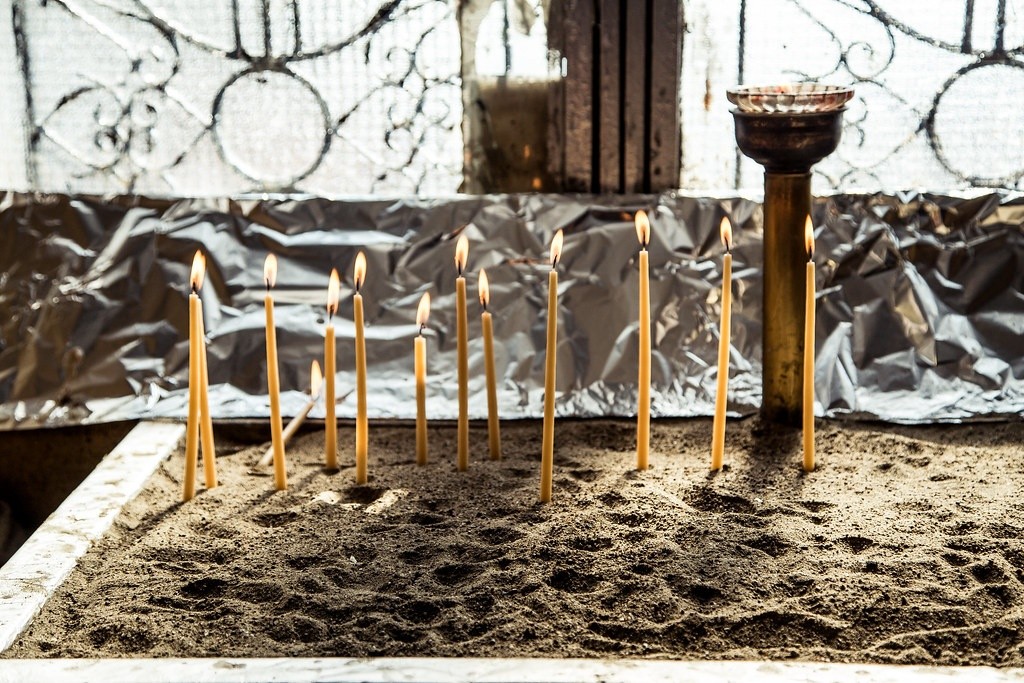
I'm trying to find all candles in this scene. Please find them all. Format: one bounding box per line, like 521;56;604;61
323;268;342;474
257;361;321;467
264;254;287;492
197;258;221;489
350;251;371;485
634;207;652;471
477;267;506;463
452;233;474;472
413;292;431;467
183;249;203;505
541;228;567;507
801;214;816;472
709;215;733;473
